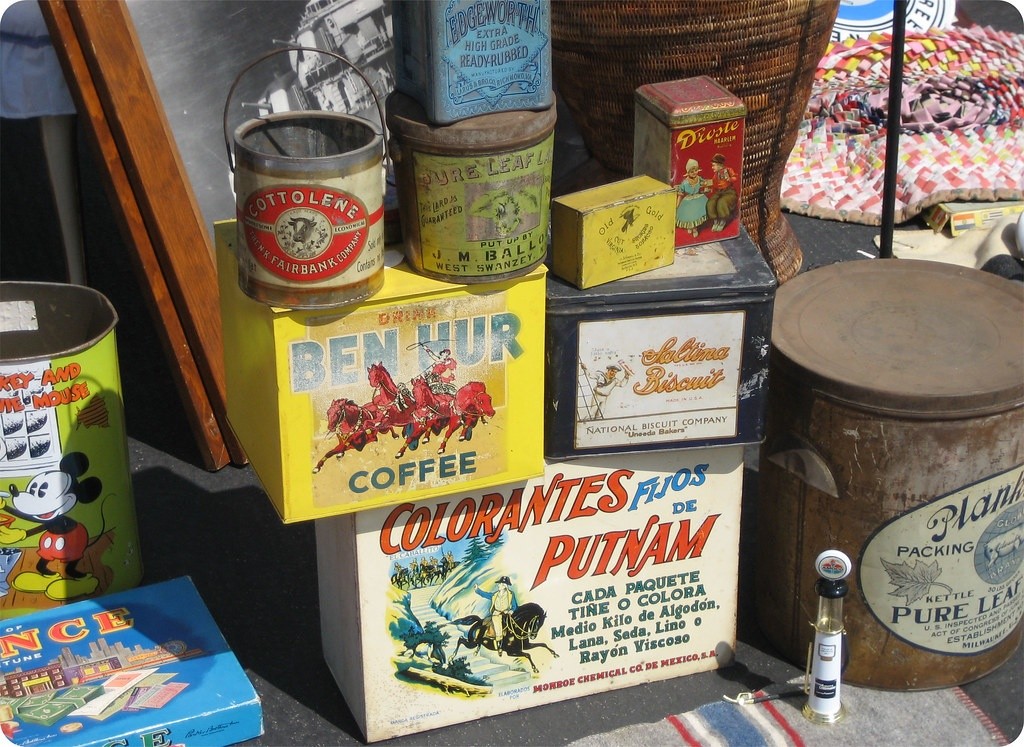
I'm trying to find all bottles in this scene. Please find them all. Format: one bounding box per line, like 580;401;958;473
800;577;847;726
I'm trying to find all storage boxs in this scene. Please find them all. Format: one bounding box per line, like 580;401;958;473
395;0;551;123
551;175;679;290
633;75;747;249
314;448;743;744
545;224;779;463
0;575;265;747
925;202;1024;238
214;219;548;525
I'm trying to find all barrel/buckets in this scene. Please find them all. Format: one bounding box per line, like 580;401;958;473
757;257;1024;692
223;47;391;314
384;92;559;281
0;278;146;623
